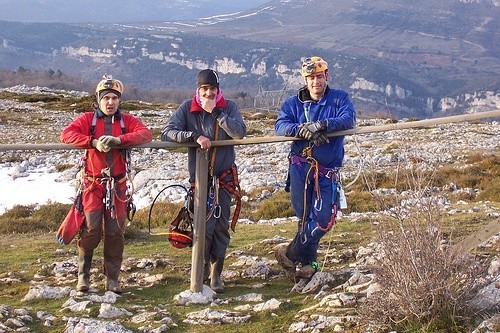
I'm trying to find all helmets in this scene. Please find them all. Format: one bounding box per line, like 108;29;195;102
96;78;124;94
301;56;328;77
169;225;193;248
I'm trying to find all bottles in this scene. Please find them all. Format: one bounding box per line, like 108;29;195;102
339;189;347;210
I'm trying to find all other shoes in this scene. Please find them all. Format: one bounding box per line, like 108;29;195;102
276;246;317;281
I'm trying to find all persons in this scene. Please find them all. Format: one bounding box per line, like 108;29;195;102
275;56;357;282
59;79;152;293
159;69;246;292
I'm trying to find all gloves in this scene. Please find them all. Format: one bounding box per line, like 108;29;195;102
298;120;330;146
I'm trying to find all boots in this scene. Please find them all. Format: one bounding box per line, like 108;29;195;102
76;255;92;291
211;256;226;292
105;277;122;293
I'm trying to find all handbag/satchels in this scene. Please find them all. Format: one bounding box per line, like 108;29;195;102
55;207;86;245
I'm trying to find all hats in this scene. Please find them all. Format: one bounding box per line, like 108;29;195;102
197;69;220;87
